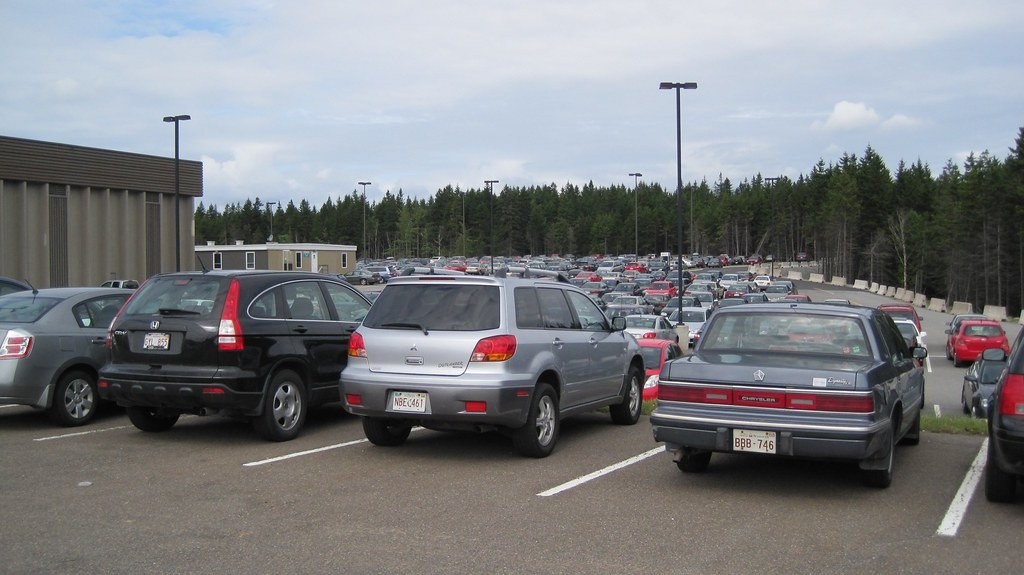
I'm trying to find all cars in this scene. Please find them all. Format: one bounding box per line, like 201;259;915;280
360;251;852;353
746;256;762;265
944;314;987;330
634;338;685;401
944;319;1010;368
766;253;775;262
649;301;927;489
960;354;1008;420
337;275;645;458
796;252;808;262
622;314;680;348
890;317;928;366
0;286;182;427
980;324;1023;503
343;269;380;286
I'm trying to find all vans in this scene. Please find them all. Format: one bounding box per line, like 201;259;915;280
98;280;140;308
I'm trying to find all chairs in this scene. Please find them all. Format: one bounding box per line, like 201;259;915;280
289;297;320;320
88;305;118;328
645;322;655;327
627;321;636;327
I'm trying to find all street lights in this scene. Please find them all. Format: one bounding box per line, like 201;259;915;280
268;202;277;240
484;180;499;274
764;177;780;280
659;81;700;354
162;115;192;273
628;173;643;263
358;181;372;265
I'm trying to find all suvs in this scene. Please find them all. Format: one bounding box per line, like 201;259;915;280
96;269;374;443
876;303;923;334
